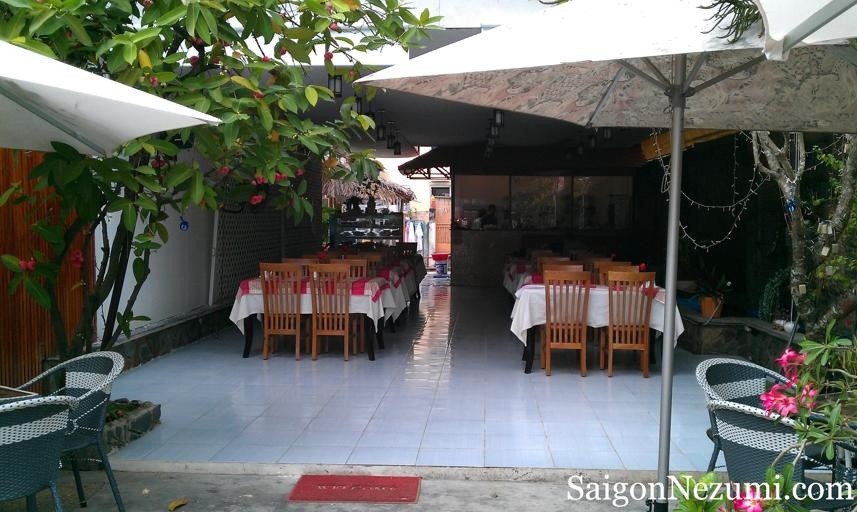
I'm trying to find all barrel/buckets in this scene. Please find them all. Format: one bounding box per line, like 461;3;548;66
434;260;447;275
699;295;725;319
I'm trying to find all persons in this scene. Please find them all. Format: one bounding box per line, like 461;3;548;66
472;209;486;230
480;205;498;229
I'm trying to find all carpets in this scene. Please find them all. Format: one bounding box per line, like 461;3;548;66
285;474;421;503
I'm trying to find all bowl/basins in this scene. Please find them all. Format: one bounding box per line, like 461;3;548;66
430;254;448;261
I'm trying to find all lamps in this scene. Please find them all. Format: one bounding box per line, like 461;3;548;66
576;127;612;155
483;110;504;160
327;73;401;155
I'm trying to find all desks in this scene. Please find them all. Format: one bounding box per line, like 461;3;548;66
502;256;686;374
242;253;425;358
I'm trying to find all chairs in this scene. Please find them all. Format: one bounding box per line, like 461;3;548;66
259;242;417;361
695;357;856;512
524;242;656;378
0;349;124;512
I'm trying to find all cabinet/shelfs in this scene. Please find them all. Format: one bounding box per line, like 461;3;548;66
330;212;405;242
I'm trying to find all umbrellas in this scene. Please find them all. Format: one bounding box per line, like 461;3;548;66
0;40;222;158
350;1;857;509
